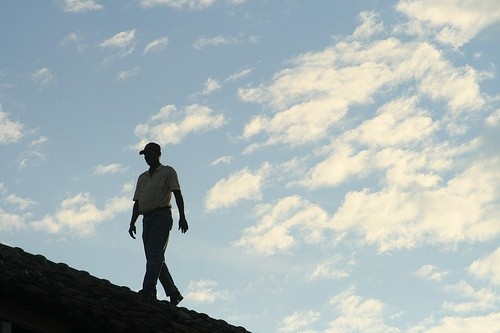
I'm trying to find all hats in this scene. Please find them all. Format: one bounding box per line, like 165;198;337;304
139;142;160;154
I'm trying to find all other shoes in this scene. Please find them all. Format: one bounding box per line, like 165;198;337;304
139;289;157;298
169;291;184;306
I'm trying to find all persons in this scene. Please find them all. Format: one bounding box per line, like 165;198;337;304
128;143;188;305
